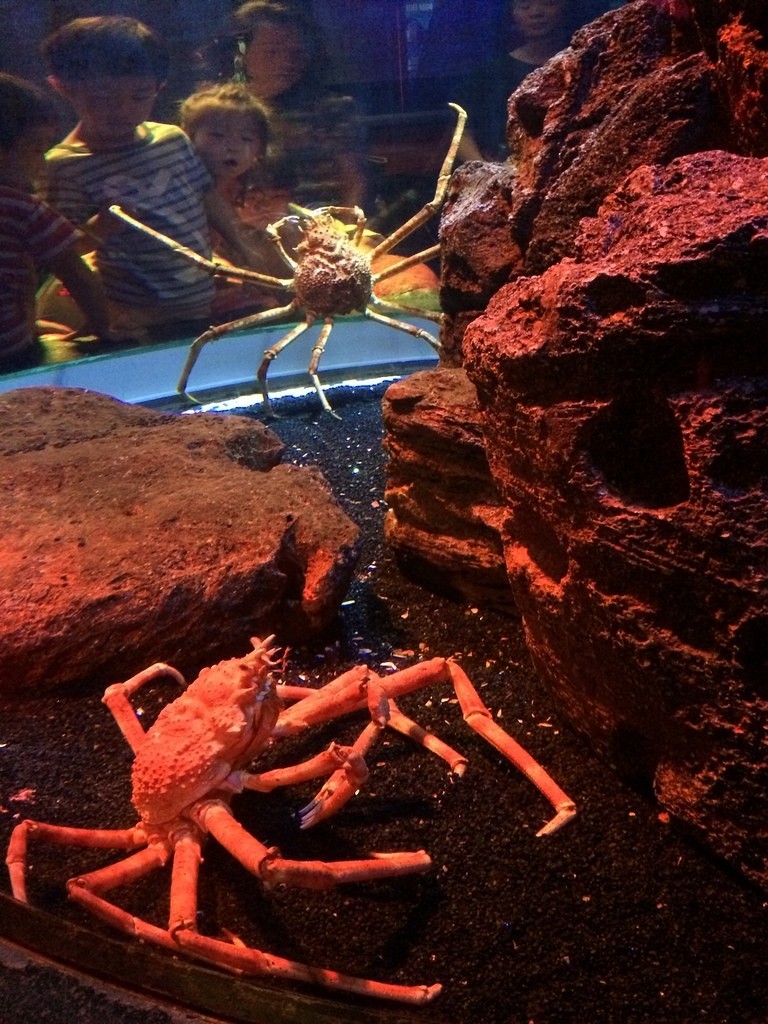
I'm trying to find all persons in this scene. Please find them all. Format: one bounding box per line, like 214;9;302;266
33;12;278;342
195;0;327;207
441;0;573;168
0;70;112;364
170;83;292;323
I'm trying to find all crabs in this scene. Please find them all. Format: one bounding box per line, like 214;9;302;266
5;635;580;1007
106;102;468;421
